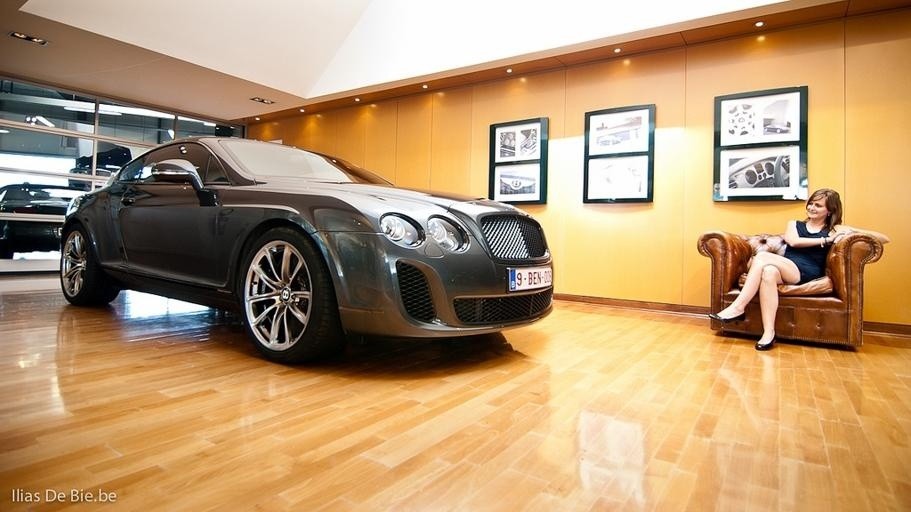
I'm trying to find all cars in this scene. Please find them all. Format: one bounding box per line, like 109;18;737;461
50;133;557;369
764;123;790;134
0;145;131;261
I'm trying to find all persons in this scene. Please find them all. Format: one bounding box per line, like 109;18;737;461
708;188;890;351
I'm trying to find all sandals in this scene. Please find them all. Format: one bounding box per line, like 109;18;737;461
755;335;776;350
709;312;745;325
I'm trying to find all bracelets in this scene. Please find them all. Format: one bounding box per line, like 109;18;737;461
825;237;827;247
820;236;824;248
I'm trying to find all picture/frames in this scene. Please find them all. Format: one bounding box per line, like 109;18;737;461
489;117;547;203
583;103;655;203
712;85;810;202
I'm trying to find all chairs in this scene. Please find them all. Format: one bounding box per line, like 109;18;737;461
696;227;883;348
